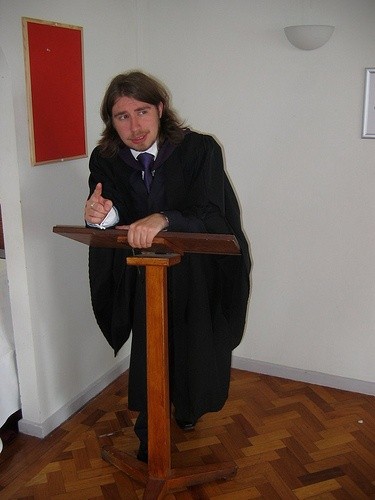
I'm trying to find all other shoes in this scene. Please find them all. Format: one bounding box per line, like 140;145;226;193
138;440;148;464
177;408;201;431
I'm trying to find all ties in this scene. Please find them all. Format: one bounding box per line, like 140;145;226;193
137;153;156;192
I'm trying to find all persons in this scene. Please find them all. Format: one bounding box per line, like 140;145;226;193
83;71;251;464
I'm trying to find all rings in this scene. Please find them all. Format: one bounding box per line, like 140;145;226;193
90;202;95;209
